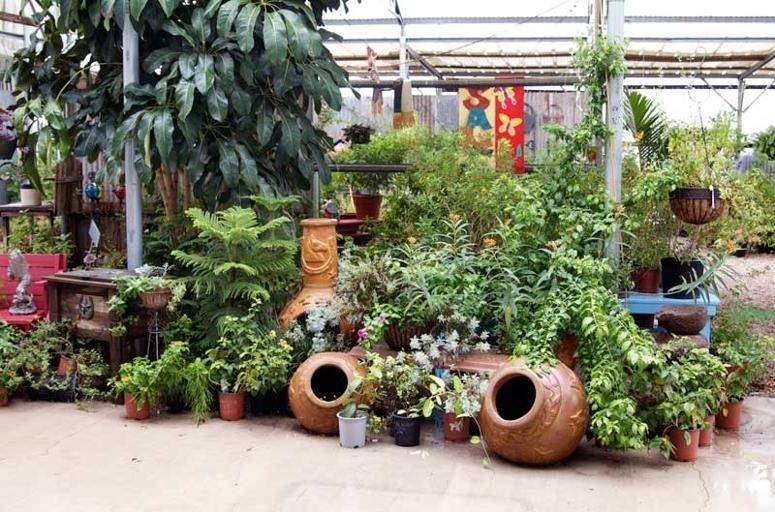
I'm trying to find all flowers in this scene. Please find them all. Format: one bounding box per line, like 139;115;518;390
362;319;491;418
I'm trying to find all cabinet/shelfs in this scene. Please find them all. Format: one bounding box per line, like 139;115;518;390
310;163;412;226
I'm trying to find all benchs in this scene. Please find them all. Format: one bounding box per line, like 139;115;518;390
1;253;65;333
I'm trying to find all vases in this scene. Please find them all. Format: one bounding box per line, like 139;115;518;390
443;411;471;440
352;195;382;220
392;412;421;446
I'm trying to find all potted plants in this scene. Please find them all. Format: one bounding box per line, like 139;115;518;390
336;404;368;447
630;247;661;293
628;148;725;224
648;292;765;463
8;164;40;206
367;254;438;352
1;316;292;419
110;271;192;314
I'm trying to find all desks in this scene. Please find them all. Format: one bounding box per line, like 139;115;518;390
42;274;160;403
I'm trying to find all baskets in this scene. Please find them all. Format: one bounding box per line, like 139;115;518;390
668;187;727;224
